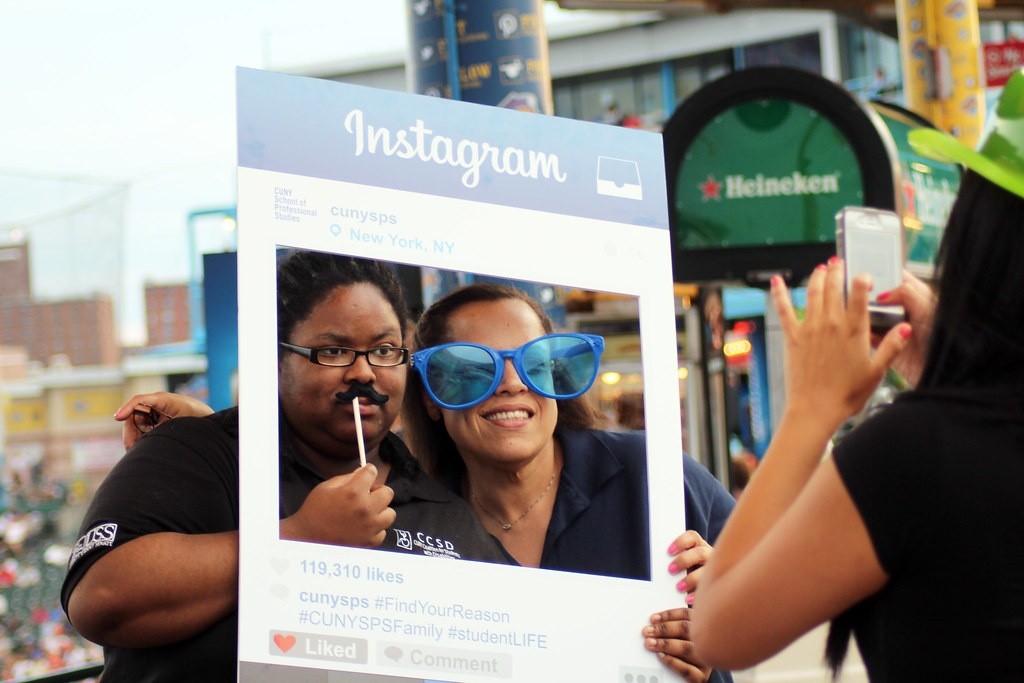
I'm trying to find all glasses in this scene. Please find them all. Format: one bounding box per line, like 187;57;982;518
410;332;604;410
279;342;409;367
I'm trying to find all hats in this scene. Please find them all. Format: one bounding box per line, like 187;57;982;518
907;67;1024;198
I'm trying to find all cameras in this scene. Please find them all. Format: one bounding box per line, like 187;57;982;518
834;206;906;328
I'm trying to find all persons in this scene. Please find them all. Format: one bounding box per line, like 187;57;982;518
690;67;1022;682
61;251;715;683
114;283;738;682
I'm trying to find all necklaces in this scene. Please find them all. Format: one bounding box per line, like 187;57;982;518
468;465;560;531
